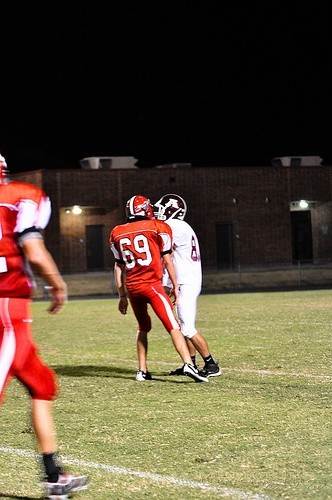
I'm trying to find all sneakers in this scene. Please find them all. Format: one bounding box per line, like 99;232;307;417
44;472;93;500
200;364;222;378
183;362;208;383
169;364;198;376
136;370;153;382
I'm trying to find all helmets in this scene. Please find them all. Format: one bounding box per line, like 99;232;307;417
0;154;10;182
153;194;187;220
126;195;153;222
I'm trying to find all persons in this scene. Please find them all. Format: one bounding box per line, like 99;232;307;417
108;195;209;382
153;194;222;378
0;154;91;500
